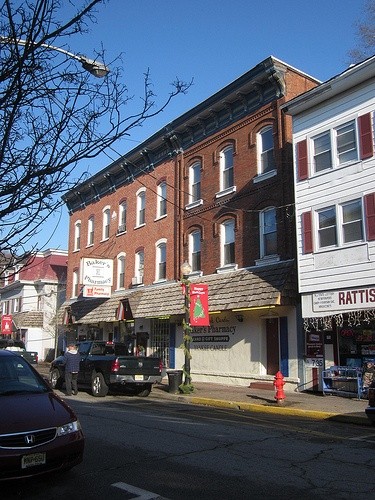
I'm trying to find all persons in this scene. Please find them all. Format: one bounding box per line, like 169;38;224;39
0;336;25;349
62;344;80;397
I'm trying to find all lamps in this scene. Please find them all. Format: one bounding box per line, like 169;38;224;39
235;315;243;322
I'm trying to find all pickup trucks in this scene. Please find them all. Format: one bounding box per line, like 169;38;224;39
0;338;37;368
50;340;163;397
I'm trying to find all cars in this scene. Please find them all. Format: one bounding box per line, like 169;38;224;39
0;351;85;482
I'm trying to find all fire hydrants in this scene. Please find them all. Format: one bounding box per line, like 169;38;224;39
273;371;287;405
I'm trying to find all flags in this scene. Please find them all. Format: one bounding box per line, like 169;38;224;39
190;283;211;327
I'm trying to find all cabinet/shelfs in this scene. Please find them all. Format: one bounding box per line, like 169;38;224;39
321;367;363;400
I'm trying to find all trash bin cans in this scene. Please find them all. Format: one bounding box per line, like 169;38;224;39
167;370;184;391
